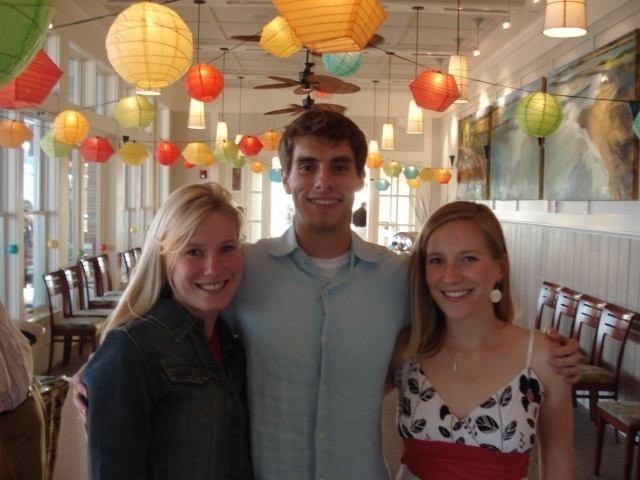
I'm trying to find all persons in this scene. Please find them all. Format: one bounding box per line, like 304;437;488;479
352;202;369;237
69;109;582;480
381;198;576;480
83;183;254;480
0;306;51;479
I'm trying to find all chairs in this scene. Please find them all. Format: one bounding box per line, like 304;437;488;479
39;247;144;373
536;280;638;478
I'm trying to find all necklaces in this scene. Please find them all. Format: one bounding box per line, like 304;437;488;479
439;316;513;374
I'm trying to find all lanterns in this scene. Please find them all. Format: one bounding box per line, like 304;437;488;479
2;0;562;189
